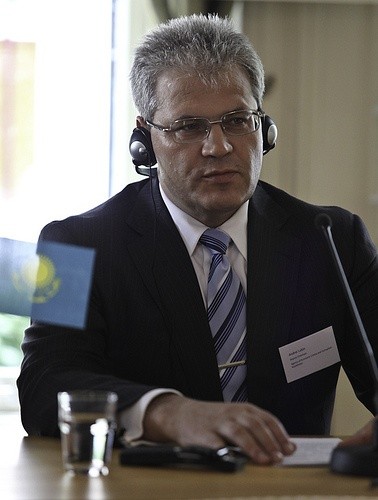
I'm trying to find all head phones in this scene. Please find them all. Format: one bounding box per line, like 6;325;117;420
129;107;278;178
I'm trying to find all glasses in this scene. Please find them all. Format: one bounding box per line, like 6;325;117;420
144;108;266;145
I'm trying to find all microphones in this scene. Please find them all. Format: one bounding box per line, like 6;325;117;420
315;214;378;477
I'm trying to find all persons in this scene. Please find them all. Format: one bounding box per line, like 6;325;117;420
16;12;378;465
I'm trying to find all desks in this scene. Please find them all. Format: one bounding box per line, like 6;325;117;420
0;437;378;500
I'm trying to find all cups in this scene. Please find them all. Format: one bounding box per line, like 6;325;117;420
57;390;119;476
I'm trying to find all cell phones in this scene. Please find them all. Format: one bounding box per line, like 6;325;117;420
119;444;245;473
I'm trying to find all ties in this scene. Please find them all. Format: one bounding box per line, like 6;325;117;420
198;228;251;404
